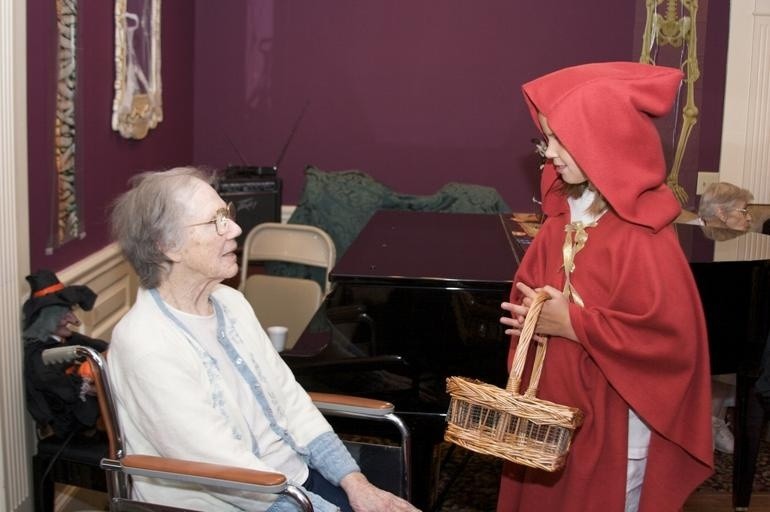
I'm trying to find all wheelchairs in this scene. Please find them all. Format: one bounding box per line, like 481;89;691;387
43;344;413;512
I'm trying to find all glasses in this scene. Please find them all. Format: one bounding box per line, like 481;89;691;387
728;208;752;215
182;200;237;236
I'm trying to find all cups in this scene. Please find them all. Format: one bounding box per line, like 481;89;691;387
266;326;290;355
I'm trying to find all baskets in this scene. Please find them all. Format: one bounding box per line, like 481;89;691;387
441;290;585;473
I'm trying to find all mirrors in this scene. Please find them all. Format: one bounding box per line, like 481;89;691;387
106;0;164;141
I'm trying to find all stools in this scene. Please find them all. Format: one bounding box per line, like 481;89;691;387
31;425;112;512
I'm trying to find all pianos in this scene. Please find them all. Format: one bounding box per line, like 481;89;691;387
291;210;769;510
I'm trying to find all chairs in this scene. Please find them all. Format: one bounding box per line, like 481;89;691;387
238;220;341;367
271;302;425;418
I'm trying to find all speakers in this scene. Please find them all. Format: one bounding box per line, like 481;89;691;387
214;176;282;245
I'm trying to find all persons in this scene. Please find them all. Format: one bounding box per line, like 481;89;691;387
493;61;717;511
684;180;755;455
20;270;108;441
105;165;422;512
